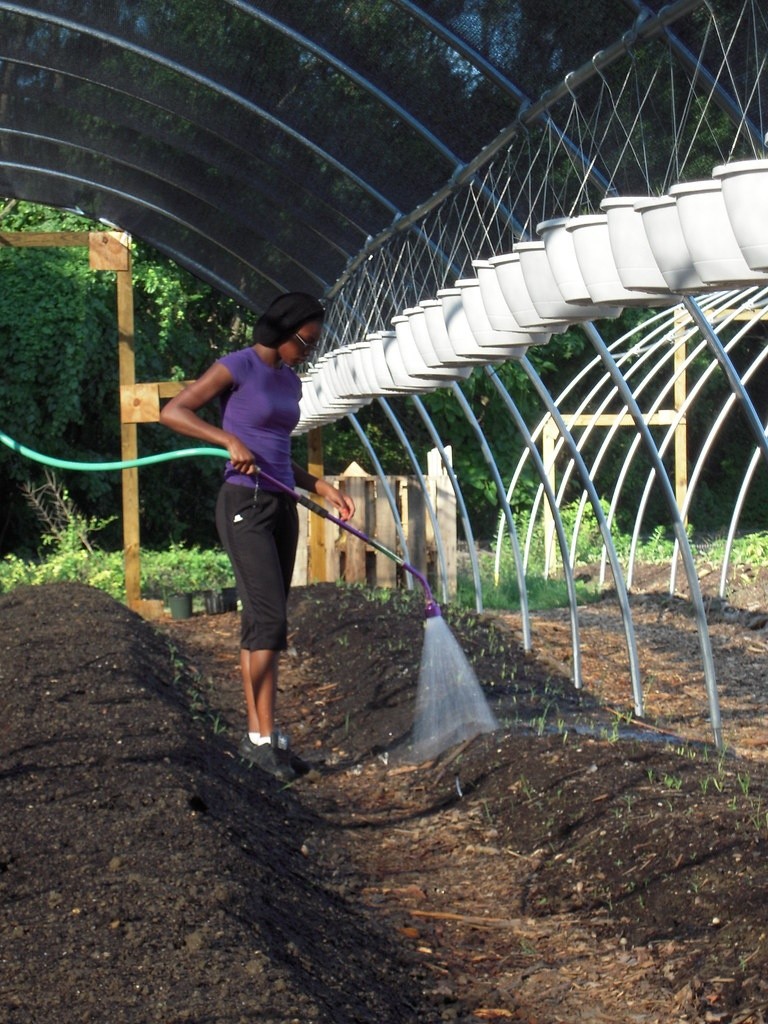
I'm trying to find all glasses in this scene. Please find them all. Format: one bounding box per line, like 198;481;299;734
295;332;320;354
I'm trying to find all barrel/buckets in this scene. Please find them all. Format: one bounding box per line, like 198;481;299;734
168;592;192;619
203;588;238;615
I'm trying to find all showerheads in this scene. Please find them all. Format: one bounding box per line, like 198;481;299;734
422;594;442;619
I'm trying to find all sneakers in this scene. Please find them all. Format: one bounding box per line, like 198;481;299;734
250;731;297;784
237;732;312;776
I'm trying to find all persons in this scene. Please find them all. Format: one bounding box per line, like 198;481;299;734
158;291;355;782
